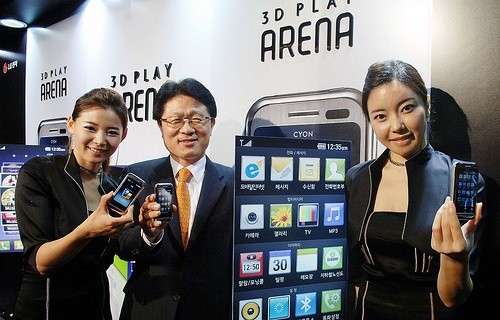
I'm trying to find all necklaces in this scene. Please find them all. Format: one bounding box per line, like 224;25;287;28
79;166;102;178
389;158;405;166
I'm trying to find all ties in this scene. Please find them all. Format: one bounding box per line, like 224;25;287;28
176;168;193;254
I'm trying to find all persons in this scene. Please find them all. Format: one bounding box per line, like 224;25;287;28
346;60;485;320
14;87;134;320
117;78;234;320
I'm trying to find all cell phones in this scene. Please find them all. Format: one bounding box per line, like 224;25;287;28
107;172;146;215
245;88;378;166
154;183;174;221
451;161;478;220
38;117;70;148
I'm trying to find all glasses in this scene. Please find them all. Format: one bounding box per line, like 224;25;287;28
160;116;212;128
